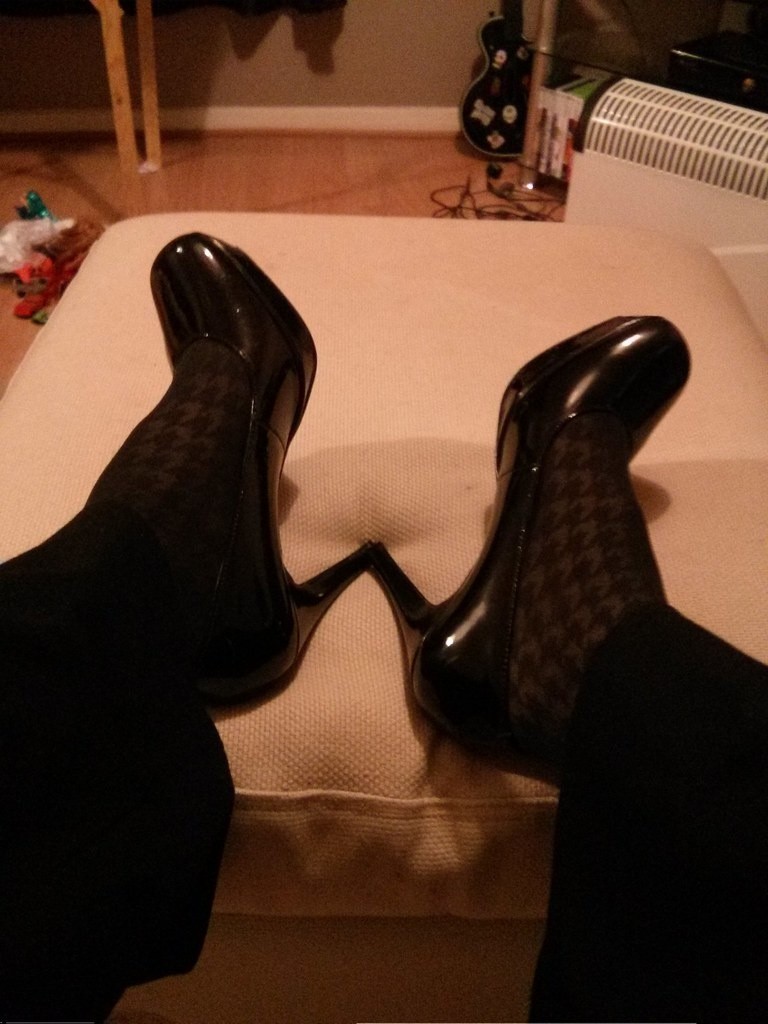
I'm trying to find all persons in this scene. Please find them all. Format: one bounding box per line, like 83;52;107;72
0;228;768;1024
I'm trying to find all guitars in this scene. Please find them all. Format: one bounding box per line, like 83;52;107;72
458;0;537;160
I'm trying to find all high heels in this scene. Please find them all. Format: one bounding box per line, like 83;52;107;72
367;315;692;785
149;231;367;717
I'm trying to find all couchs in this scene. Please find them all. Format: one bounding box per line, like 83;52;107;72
0;210;767;1023
